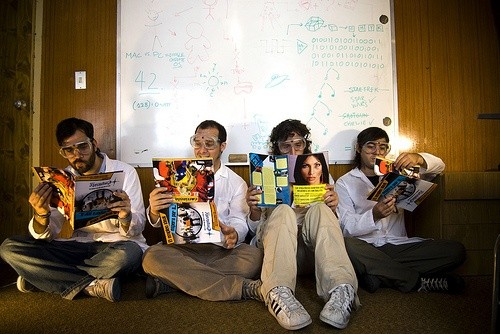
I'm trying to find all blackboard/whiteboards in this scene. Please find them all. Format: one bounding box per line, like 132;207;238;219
116;0;400;168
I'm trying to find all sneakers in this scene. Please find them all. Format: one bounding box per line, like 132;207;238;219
268;286;313;330
319;283;355;329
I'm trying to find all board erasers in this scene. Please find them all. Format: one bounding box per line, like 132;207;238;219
228;154;247;163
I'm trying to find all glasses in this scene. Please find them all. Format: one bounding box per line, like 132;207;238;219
58;140;92;158
190;135;221;150
362;140;392;157
275;138;307;154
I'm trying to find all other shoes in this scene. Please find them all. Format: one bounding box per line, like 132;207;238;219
417;272;465;295
144;277;178;297
358;274;380;293
16;275;45;293
84;278;122;303
244;278;265;302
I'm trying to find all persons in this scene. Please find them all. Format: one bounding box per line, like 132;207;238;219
245;119;358;330
0;117;150;302
142;120;265;303
294;153;329;184
334;127;468;293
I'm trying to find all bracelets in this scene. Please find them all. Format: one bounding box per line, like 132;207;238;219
118;211;132;222
34;209;51;218
150;210;159;216
